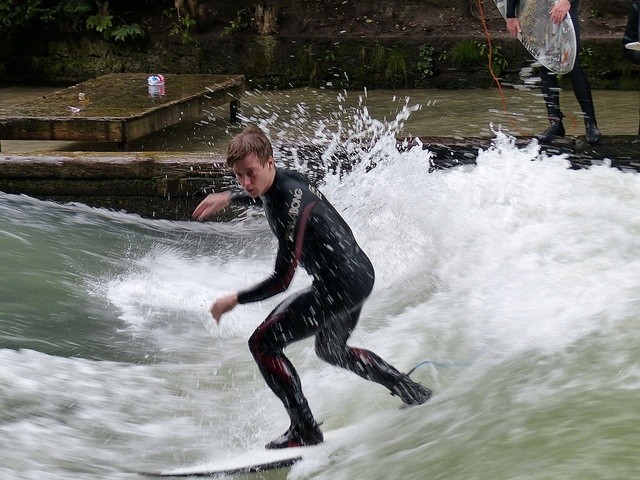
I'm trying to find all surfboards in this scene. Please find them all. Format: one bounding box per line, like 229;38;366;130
139;446;302;477
494;0;577;75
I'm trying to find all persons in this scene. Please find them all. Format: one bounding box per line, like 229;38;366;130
193;125;432;449
506;0;601;143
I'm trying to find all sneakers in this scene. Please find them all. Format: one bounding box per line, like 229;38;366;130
539;121;565;142
265;422;323;449
393;377;433;409
585;118;601;143
